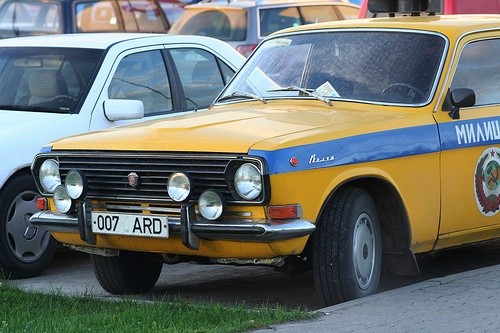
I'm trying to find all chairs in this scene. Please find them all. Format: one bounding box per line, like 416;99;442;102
182;59;221;108
28;69;68;106
110;59;161;109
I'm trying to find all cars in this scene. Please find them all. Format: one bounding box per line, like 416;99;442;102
0;33;290;285
27;12;500;307
0;0;500;85
